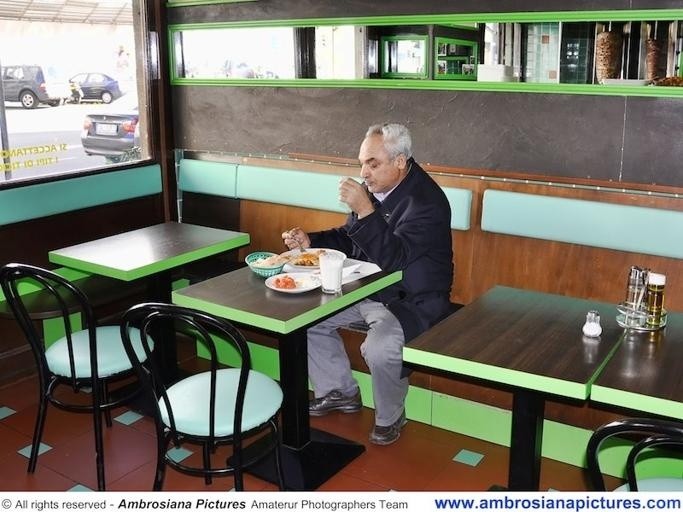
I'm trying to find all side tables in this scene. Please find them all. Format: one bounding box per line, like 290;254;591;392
46;219;250;421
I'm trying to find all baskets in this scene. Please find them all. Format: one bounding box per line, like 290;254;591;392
245;250;286;279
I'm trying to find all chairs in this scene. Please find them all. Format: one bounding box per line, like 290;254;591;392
0;261;163;491
117;301;297;491
584;416;682;491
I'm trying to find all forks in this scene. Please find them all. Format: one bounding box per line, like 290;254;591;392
290;238;307;255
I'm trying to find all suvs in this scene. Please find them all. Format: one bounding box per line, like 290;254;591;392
0;64;71;109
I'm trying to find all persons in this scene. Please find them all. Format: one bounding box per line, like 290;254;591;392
280;123;454;447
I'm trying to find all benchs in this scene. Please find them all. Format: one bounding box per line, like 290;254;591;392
433;174;683;482
171;147;477;431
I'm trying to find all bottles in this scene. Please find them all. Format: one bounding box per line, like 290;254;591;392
620;330;663;379
582;310;603;338
624;265;666;328
583;339;601;369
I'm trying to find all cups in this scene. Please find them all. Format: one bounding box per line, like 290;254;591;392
317;252;345;295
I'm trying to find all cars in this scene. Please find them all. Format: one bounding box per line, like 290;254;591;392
66;69;125;105
82;90;140;163
150;52;281;81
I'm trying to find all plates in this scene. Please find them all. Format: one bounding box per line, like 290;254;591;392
603;78;650;85
475;63;513;84
263;273;321;293
280;247;347;270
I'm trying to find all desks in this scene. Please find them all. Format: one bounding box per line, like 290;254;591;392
170;247;403;491
401;284;640;491
585;309;681;492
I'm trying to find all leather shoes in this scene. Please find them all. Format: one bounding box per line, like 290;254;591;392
307;385;363;418
368;406;408;446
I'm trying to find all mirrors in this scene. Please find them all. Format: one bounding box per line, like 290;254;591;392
377;32;428;80
165;9;683;98
432;37;478;81
